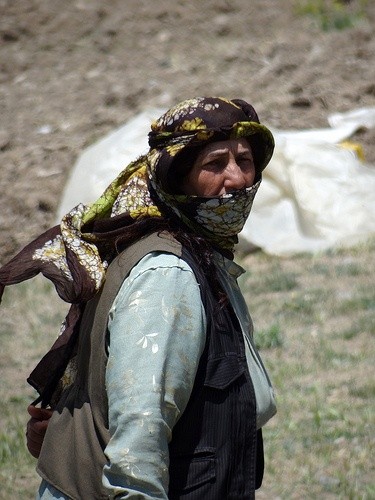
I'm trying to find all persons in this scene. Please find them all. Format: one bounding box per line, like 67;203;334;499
1;99;278;500
